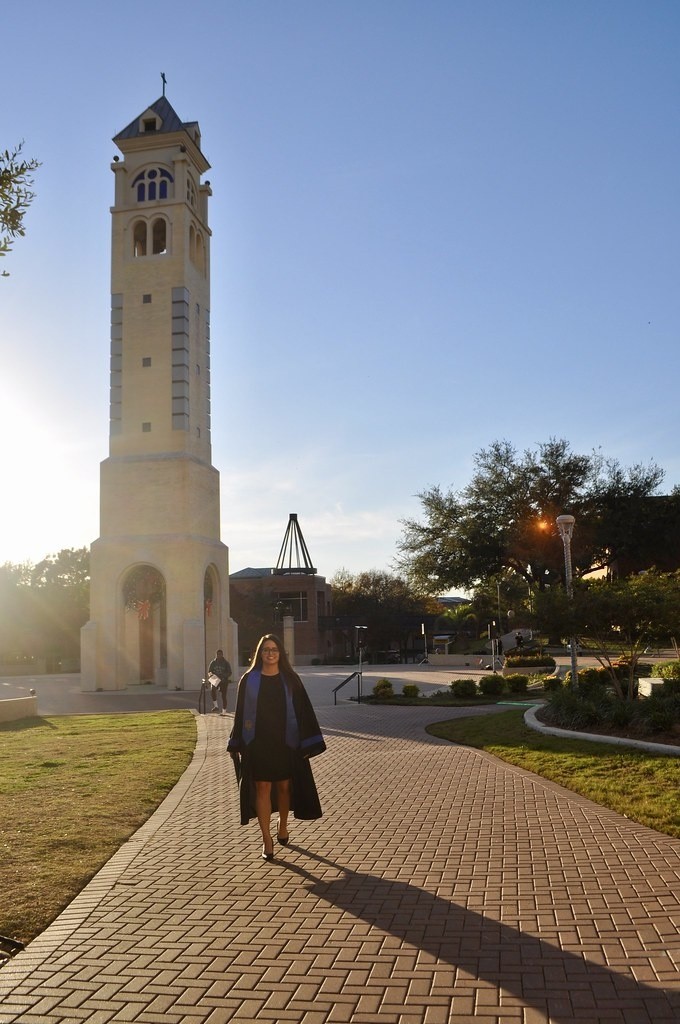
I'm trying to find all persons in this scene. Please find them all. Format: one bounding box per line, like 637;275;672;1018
516;632;524;647
208;650;231;716
227;633;327;861
498;637;502;655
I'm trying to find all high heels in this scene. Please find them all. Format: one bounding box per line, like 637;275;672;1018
262;837;273;861
276;821;289;845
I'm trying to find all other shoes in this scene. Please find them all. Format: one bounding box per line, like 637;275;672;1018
211;707;219;711
219;713;226;716
